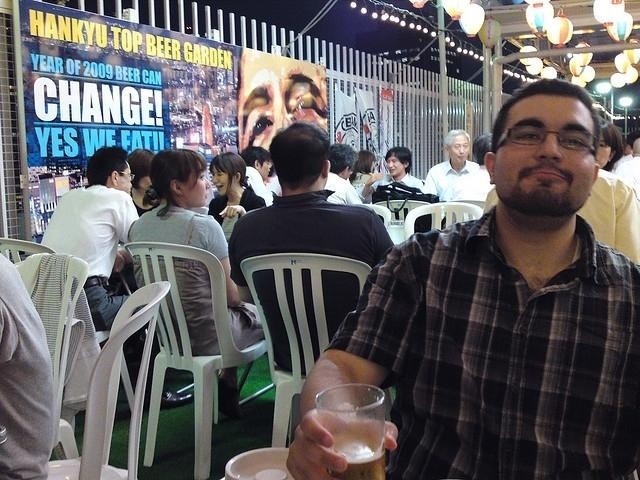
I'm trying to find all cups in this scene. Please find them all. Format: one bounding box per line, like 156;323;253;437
314;383;386;480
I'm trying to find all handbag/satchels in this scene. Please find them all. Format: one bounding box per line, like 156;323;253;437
371;183;439;232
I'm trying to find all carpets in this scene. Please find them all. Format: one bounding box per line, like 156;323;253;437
50;353;275;479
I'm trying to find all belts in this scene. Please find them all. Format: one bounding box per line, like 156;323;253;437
84;277;105;288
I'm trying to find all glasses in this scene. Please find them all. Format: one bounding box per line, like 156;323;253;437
121;171;135;181
495;126;598;151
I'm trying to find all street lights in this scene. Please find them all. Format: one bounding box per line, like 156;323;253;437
619;97;632;142
596;82;613;125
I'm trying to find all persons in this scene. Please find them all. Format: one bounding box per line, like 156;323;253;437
208;152;267;242
325;144;363;205
40;146;194;408
241;147;275;207
613;129;640;200
227;121;397;390
471;134;496;177
483;169;640;266
348;151;379;200
420;129;496;201
285;79;640;480
593;113;623;174
128;149;161;217
621;137;632;156
0;253;58;480
127;149;266;420
354;148;424;205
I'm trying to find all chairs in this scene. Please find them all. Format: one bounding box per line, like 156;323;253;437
14;252;89;459
404;202;482;238
0;238;134;414
45;280;171;480
124;243;267;480
240;253;374;447
374;200;430;225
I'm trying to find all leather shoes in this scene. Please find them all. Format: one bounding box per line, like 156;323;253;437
218;380;245;419
161;391;193;407
